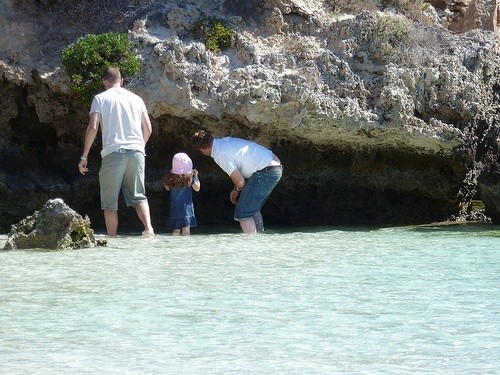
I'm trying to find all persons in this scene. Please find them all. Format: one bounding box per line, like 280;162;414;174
164;153;200;236
77;67;155;238
191;130;283;233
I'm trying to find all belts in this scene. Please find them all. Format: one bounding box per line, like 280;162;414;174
263;164;282;169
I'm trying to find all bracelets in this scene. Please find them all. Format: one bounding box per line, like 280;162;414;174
80;156;87;160
234;185;240;192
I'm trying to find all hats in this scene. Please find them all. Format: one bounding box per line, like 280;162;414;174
170;153;195;175
